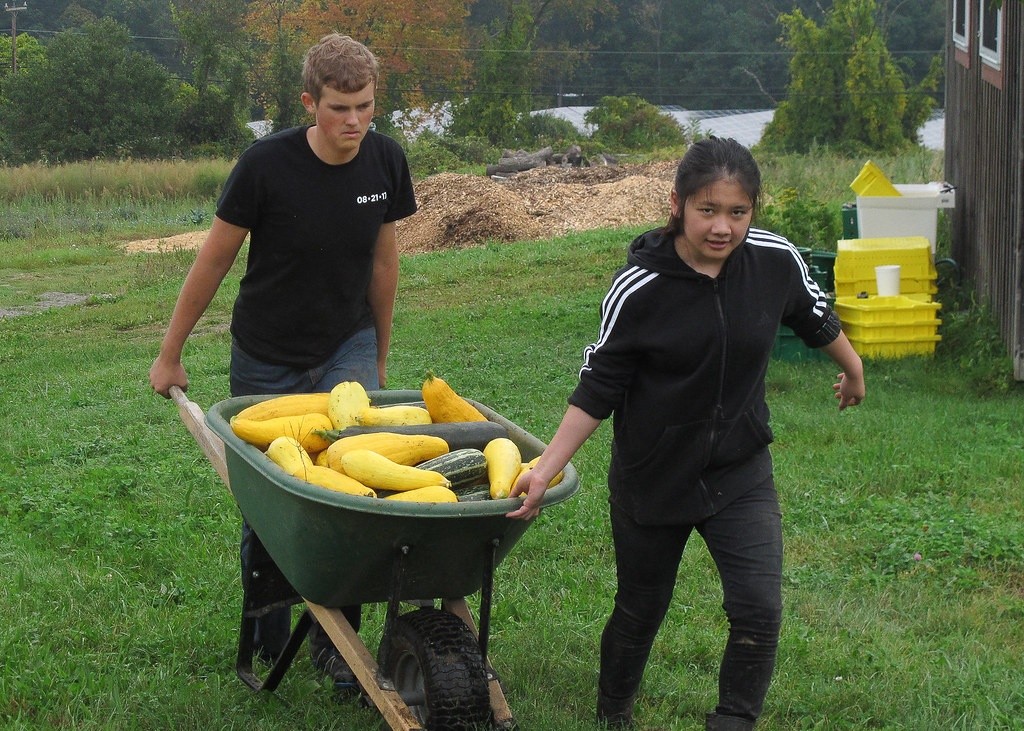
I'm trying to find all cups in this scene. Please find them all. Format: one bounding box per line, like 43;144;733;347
874;265;901;296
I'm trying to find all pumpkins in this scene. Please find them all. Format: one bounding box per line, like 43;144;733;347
230;369;564;504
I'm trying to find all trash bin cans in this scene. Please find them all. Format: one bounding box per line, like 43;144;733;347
840;200;859;240
857;180;956;266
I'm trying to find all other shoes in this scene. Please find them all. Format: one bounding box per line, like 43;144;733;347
311;645;357;687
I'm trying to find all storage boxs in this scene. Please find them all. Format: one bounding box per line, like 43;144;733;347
775;247;836;360
832;204;942;362
849;159;902;196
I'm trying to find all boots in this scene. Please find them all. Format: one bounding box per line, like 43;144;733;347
596;684;638;731
704;713;753;730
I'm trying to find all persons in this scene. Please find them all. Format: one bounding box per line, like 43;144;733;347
147;34;418;702
506;134;866;731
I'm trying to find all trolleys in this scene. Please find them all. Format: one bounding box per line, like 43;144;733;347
168;380;579;731
